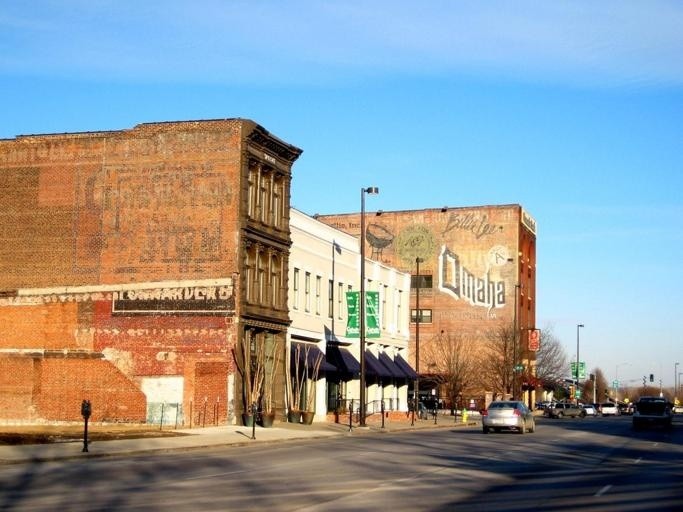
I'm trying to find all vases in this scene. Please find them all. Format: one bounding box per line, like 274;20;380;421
289;410;314;424
241;413;275;428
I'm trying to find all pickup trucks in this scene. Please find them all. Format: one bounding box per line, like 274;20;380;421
544;402;587;419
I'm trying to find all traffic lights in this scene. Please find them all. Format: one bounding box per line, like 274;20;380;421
650;373;653;382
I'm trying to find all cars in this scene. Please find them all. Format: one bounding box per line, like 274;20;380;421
632;396;674;432
600;401;621;416
619;403;634;415
481;400;536;433
582;404;597;416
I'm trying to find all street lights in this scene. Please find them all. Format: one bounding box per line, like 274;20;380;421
414;256;425;411
512;282;522;401
575;323;584;406
355;185;381;433
674;363;683;392
